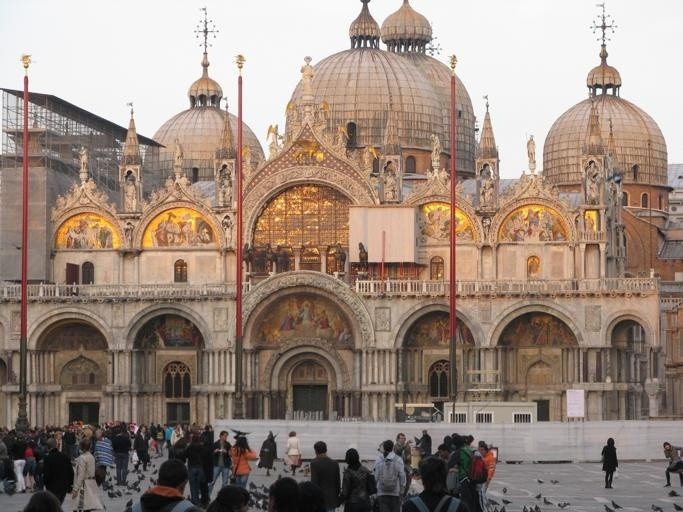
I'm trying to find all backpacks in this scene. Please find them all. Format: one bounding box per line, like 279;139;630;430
458;447;488;484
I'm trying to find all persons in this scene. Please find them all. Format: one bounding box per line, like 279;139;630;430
663;441;683;488
426;205;473;241
260;430;498;512
64;218;114;249
506;208;565;241
253;298;352;343
527;257;539;274
1;422;256;512
241;242;253;263
515;320;567;345
153;216;211;246
584;214;594;231
139;321;203;347
599;435;619;489
434;315;465;345
